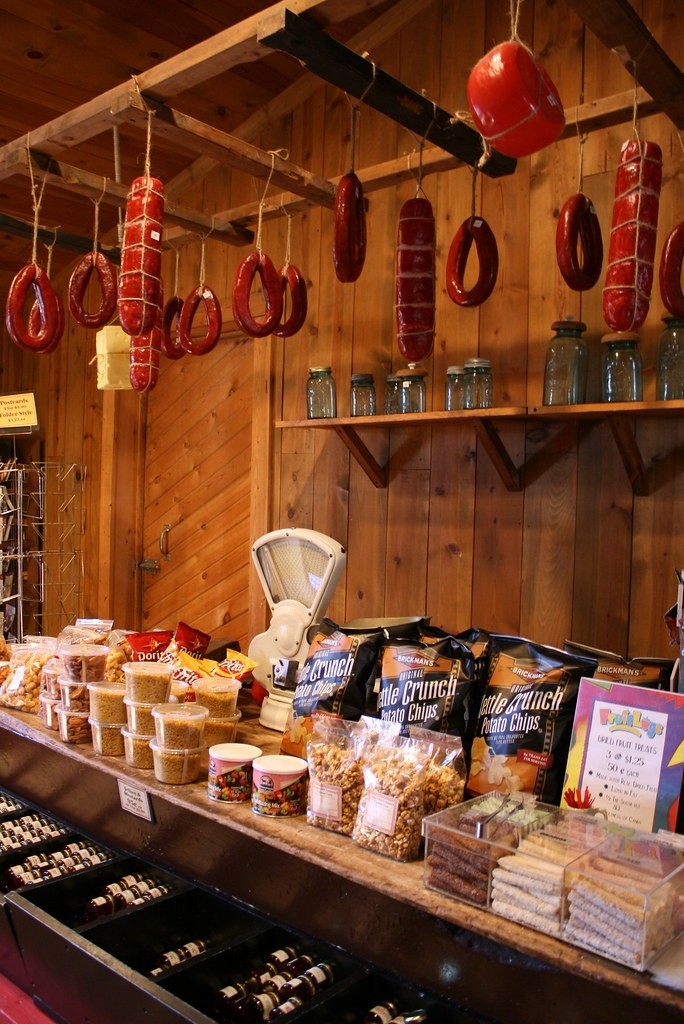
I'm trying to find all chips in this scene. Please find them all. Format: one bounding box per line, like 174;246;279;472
469;736;525;794
282;703;312;760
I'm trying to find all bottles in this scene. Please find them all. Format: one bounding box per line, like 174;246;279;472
350;373;377;417
306;366;337;421
654;311;684;402
541;320;591;406
444;357;493;411
600;332;643;404
383;368;429;415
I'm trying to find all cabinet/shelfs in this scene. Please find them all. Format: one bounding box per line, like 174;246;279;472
0;706;684;1024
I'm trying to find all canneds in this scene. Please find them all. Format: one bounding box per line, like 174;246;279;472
0;796;432;1024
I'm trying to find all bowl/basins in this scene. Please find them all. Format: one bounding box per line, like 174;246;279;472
40;642;241;785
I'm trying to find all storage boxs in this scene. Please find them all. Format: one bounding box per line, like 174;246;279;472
422;791;684;971
